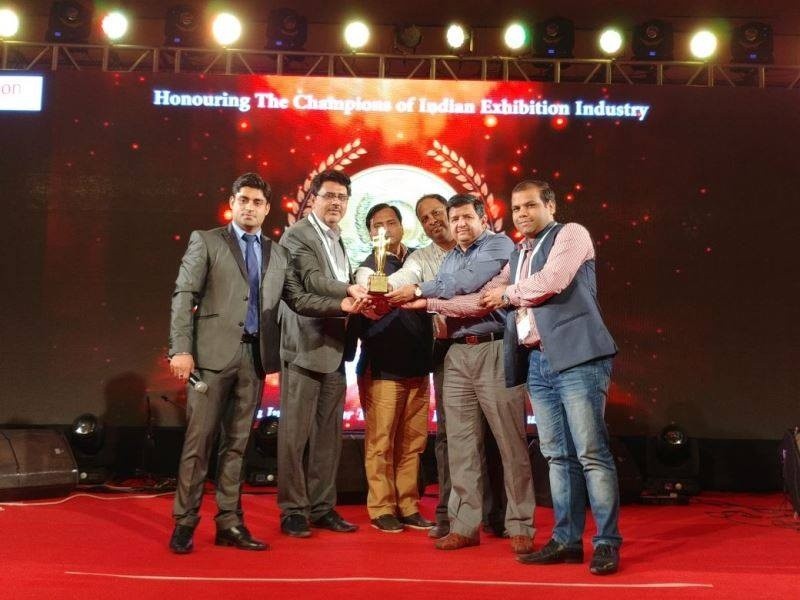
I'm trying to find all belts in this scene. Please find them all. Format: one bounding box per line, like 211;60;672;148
238;332;260;345
449;330;507;345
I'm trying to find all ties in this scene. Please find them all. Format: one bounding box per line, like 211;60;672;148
238;232;262;336
328;230;349;286
518;249;534;320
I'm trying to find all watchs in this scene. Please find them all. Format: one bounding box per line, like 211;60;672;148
414;284;424;300
501;293;511;306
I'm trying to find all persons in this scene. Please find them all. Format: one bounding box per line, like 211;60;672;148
160;169;377;558
398;178;627;578
366;194;509;540
349;203;437;534
276;170;370;533
386;194;546;555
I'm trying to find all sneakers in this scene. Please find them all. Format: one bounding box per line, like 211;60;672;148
400;511;439;532
370;511;404;533
428;518;450;540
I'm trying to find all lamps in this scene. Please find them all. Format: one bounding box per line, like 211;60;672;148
160;4;207;71
728;20;773;89
389;21;423;65
44;1;95;56
70;412;277;488
631;18;677;86
525;8;577;83
528;425;701;506
261;7;309;65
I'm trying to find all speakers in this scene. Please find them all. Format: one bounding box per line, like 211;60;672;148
300;437;424;505
0;428;78;503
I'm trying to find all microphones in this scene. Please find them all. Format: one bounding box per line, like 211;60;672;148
164;352;208;394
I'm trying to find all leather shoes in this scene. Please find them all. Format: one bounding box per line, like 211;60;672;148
509;532;537;555
516;538;588;566
433;530;482;550
168;522;198;553
315;509;359;534
280;513;314;538
210;526;266;550
590;542;621;575
